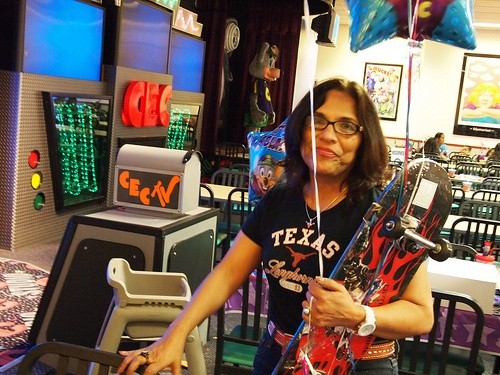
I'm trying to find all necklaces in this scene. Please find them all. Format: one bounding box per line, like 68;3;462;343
306;186;346;227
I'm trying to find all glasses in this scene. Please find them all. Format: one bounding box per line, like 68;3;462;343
305;115;365;136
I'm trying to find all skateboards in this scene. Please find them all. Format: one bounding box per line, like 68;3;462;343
270;157;453;375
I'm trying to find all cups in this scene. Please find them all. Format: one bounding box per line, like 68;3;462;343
462;181;473;191
476;253;495;264
448;169;457;177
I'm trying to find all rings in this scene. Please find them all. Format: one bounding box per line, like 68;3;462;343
140;350;150;362
304;305;310;314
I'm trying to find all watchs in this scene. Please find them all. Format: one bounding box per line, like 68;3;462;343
348;305;376;336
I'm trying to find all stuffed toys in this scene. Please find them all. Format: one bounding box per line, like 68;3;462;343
244;42;284;149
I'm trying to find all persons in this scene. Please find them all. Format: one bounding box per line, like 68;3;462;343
419;132;471;160
483;143;500;168
118;78;434;375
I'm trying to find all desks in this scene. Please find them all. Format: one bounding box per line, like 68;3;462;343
228;152;249;159
449;175;500;186
442;215;500;244
452;190;500;201
214;255;500;375
201;183;249;205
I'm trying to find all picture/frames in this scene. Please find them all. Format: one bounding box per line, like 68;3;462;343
363;63;403;121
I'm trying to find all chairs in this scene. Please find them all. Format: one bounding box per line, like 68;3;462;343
17;258;207;374
200;145;500;375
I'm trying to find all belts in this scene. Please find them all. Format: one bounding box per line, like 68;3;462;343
267;321;395;360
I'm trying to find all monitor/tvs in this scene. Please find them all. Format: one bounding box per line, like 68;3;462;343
311;8;340;47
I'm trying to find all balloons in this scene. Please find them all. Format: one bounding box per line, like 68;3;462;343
247;117;287;202
348;0;477;50
